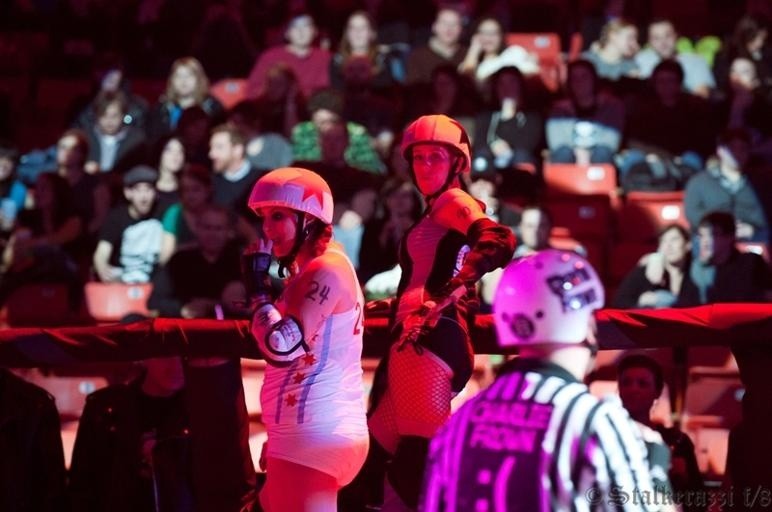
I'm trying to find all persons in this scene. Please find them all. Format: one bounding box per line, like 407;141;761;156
2;1;772;511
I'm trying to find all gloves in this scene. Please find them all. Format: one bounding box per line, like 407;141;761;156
238;252;273;299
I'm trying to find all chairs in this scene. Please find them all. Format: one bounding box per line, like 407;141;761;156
1;30;771;473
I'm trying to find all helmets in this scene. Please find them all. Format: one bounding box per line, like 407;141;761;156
248;166;334;224
492;249;606;349
401;114;471;174
